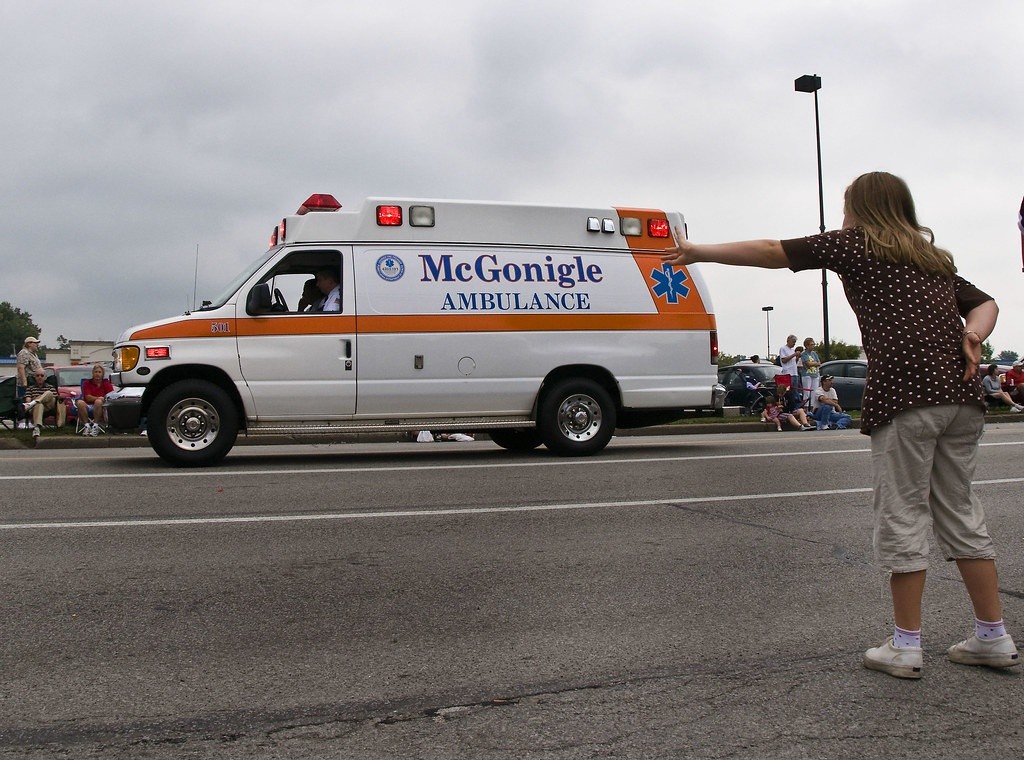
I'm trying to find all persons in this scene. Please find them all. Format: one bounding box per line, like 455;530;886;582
747;335;852;432
22;369;58;438
16;336;43;429
981;361;1024;412
298;263;341;312
662;172;1020;679
76;364;114;437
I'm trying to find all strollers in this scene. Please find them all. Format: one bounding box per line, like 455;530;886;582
735;368;778;414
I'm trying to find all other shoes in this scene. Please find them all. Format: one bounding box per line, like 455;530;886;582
777;427;782;432
799;423;816;431
761;418;766;422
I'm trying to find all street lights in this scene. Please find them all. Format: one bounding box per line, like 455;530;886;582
763;307;774;360
795;74;829;344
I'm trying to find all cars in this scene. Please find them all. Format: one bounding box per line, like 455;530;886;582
41;366;114;427
819;359;868;411
716;364;783;412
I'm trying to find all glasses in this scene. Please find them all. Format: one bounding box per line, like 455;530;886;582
36;374;45;377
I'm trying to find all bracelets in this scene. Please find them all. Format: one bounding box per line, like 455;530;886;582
962;330;982;345
97;397;98;399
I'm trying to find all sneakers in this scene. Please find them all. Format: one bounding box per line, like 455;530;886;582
863;636;923;680
82;426;99;437
32;428;41;438
1009;403;1024;413
820;425;830;431
22;403;31;412
947;633;1020;668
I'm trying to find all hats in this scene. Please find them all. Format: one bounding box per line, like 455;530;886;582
821;374;834;381
25;337;41;344
1012;361;1023;367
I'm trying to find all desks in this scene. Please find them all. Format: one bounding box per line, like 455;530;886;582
747;387;785;416
1001;385;1017;410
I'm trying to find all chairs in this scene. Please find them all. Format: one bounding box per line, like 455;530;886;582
0;376;59;434
774;373;813;413
76;379;110;435
984;395;1002;414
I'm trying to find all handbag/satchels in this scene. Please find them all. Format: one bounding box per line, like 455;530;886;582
55;399;66;428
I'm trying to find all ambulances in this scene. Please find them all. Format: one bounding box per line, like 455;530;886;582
101;194;728;468
